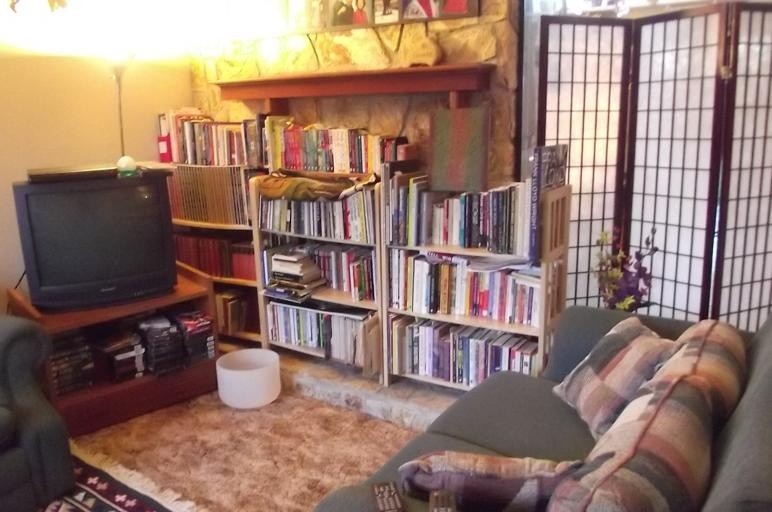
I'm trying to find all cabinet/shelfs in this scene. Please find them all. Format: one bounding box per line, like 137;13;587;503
384;169;574;393
154;161;266;354
7;263;223;437
248;161;383;387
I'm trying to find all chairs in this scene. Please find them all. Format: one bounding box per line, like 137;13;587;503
0;315;77;511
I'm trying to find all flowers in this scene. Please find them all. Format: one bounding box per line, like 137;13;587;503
585;223;662;314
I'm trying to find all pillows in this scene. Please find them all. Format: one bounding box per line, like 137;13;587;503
399;448;584;510
555;319;687;435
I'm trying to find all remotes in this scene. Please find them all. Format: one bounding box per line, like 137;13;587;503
428;488;458;512
370;480;405;512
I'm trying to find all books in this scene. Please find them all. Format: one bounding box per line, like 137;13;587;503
159;108;257;165
263;234;376;304
389;143;568;266
256;112;418;176
267;299;375;364
48;309;215;395
390;314;538;388
258;187;375;244
176;230;257;281
389;248;541;326
215;287;259;333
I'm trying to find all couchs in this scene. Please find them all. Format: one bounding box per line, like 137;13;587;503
311;304;772;511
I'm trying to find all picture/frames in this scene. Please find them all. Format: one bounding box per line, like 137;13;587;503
327;0;479;32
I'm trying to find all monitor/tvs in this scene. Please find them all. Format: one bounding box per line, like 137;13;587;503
12;172;179;311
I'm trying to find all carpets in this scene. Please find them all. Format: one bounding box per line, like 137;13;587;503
40;441;203;512
